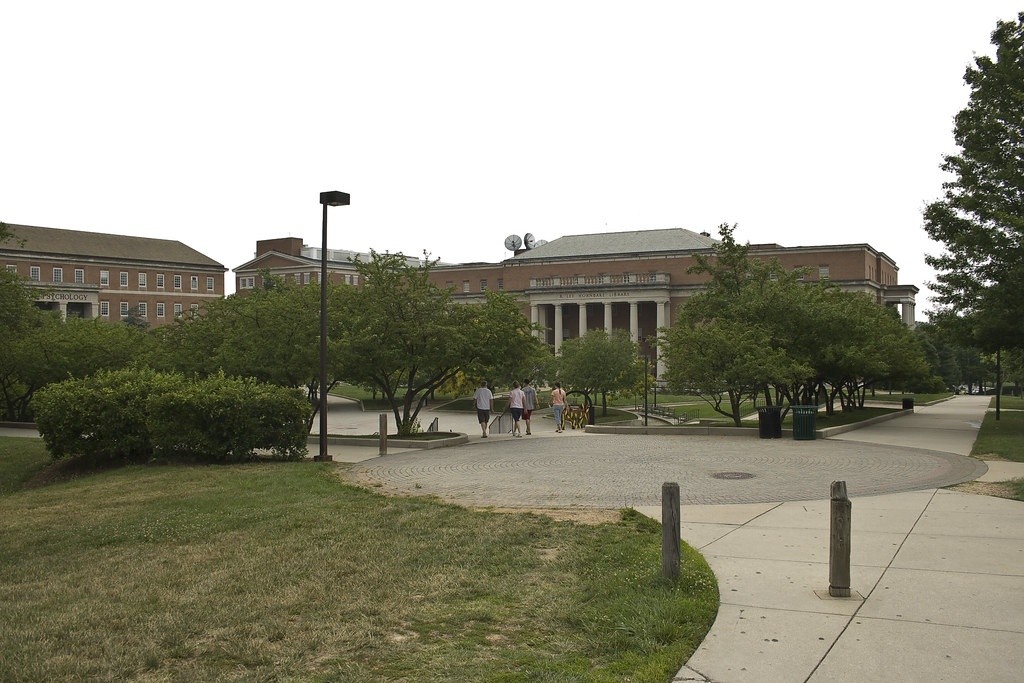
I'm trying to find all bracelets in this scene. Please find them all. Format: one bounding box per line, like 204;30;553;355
536;404;539;405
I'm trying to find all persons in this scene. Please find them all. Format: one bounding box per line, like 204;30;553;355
507;380;527;436
473;381;494;438
548;382;567;433
522;379;540;435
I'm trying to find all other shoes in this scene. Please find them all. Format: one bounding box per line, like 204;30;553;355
526;430;531;435
556;429;563;433
513;432;522;437
481;434;488;438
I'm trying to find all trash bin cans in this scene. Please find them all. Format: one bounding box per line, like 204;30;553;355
754;405;786;439
789;404;819;440
902;397;914;412
